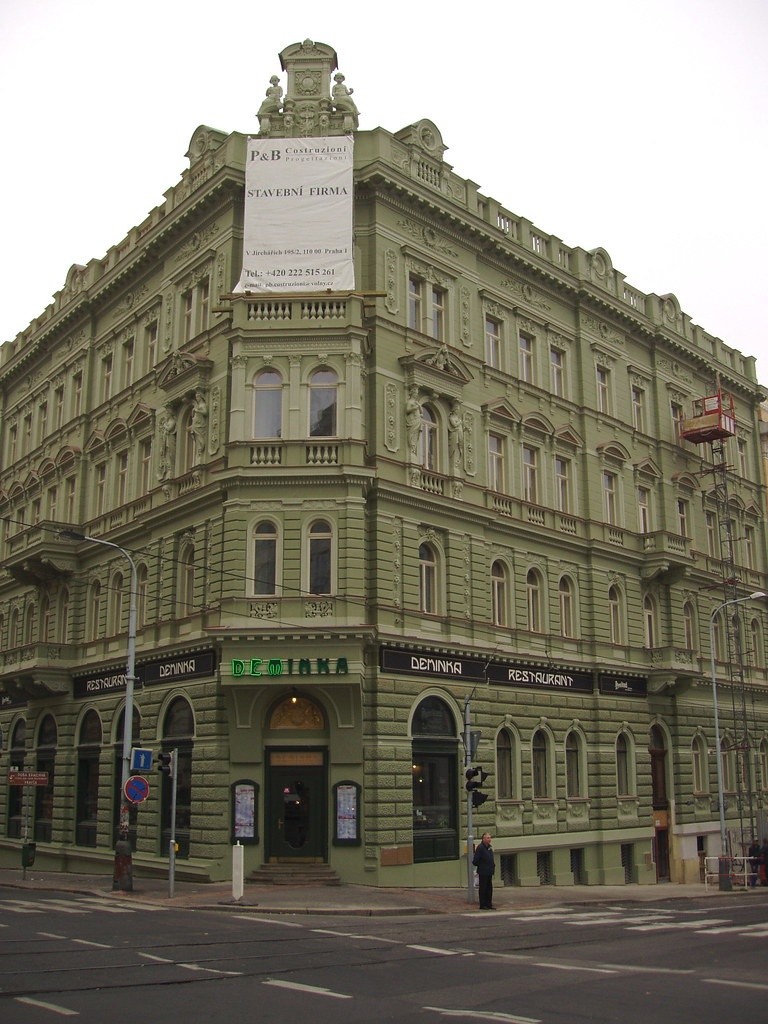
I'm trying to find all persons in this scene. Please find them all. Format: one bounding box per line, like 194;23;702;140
749;837;768;889
472;832;497;910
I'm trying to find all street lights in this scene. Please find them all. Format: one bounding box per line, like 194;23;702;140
56;527;134;896
707;591;768;853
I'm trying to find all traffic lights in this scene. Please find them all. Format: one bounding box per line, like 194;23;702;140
473;791;489;808
465;766;479;793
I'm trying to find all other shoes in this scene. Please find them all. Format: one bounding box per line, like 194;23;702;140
480;905;496;910
